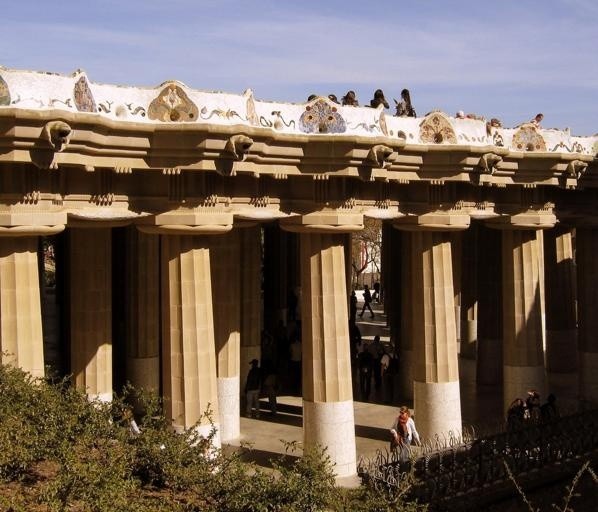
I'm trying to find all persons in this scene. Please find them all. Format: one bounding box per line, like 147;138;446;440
263;373;277;416
505;390;561;432
390;406;422;462
531;113;543;129
245;359;261;420
350;282;398;404
306;88;415;119
